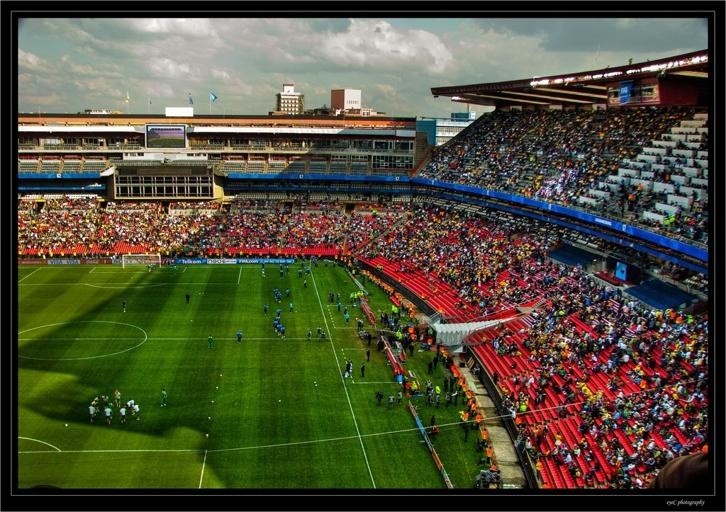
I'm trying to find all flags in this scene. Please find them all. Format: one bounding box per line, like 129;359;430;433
208;91;218;103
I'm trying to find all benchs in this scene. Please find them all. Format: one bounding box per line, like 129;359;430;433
18;108;712;489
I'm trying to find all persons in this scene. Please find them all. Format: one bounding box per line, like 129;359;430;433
17;109;710;489
